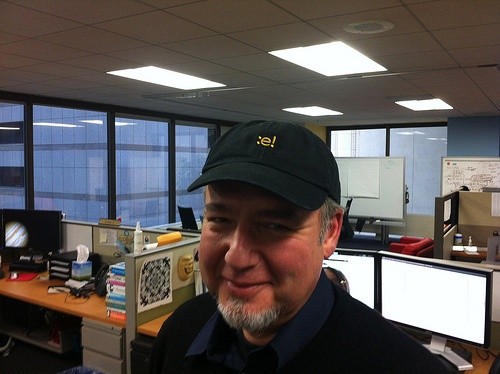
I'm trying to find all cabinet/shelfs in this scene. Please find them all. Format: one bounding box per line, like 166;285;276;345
47;249;103;283
81;316;126;374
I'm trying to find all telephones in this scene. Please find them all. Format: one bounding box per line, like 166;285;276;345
93;261;116;296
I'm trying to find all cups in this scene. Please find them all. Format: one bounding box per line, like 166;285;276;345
133;221;143;253
454;233;462;245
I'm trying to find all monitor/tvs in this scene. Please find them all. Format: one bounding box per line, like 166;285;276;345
323;249;378;311
378;250;494;371
177;205;198;230
0;209;62;271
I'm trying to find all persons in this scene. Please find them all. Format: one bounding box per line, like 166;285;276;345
144;119;456;374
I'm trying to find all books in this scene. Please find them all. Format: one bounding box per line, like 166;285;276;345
105;261;126;323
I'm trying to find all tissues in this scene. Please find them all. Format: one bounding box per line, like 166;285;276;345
71;245;92;281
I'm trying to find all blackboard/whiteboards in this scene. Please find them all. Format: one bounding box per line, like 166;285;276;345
440;155;500;223
334;155;406;222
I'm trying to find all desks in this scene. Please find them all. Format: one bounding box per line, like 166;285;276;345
137;311;500;374
450;250;500;261
0;261;127;356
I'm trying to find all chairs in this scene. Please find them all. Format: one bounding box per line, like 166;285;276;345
389;235;434;259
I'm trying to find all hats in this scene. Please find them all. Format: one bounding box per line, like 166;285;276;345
185;118;342;211
